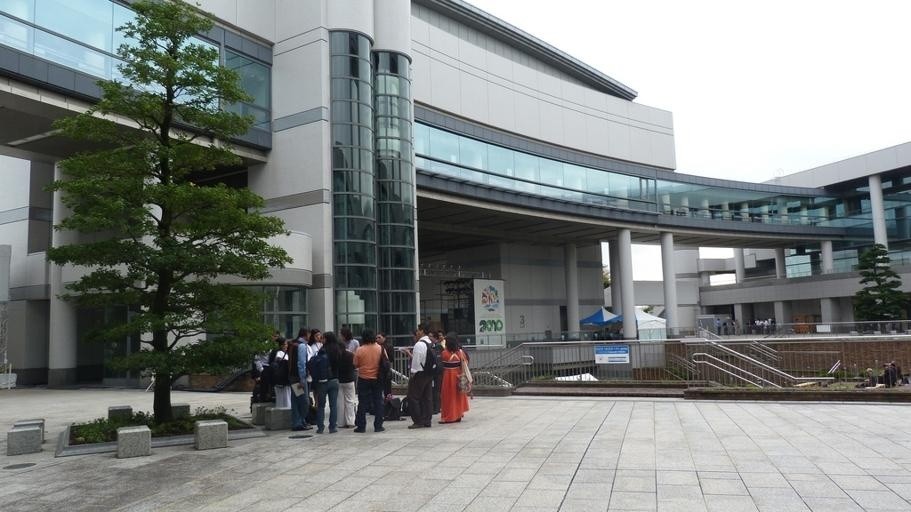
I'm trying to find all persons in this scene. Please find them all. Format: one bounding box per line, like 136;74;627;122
716;317;775;336
250;323;473;434
855;361;902;388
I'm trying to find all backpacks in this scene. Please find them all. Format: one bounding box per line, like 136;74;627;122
383;395;411;422
308;354;330;383
376;344;393;387
420;339;444;376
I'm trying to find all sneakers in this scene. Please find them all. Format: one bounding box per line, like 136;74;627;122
329;428;338;433
316;428;325;434
338;425;356;428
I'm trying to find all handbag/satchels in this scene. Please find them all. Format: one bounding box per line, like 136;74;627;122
268;361;298;385
457;374;472;395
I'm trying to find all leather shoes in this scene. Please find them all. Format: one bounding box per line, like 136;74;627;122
353;426;368;433
373;425;386;433
292;421;314;431
407;422;433;429
